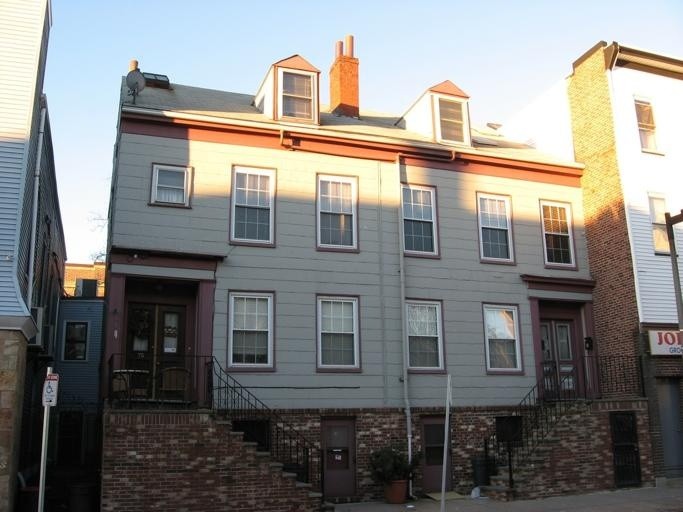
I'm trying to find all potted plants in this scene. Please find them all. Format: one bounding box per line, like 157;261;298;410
369;447;424;504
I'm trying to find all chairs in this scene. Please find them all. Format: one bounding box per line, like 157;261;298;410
109;369;151;408
156;367;192;408
